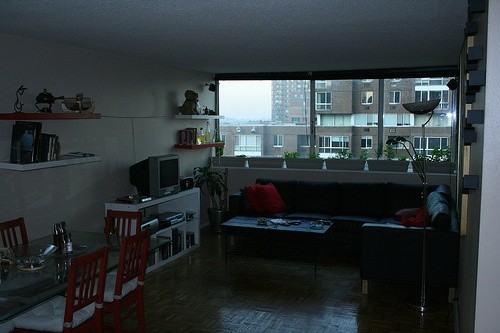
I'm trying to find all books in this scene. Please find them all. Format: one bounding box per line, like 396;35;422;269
186;232;195;248
172;229;184;256
10;121;60;165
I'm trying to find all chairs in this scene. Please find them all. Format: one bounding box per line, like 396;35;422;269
13;247;108;333
67;228;151;333
106;210;142;237
0;217;28;247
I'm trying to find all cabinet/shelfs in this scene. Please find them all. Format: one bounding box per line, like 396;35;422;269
174;114;225;149
105;187;202;274
0;111;102;172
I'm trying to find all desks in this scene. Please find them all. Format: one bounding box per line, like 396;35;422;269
0;230;172;333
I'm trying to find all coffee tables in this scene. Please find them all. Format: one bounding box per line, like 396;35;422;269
220;216;335;277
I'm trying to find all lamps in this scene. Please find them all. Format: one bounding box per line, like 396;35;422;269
385;136;424;186
447;77;459;90
205;83;217;92
402;98;442;313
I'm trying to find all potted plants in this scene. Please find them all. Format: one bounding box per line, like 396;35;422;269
412;146;455;173
284;152;323;169
325;152;367;169
367;145;409;172
193;164;229;235
209;154;246;168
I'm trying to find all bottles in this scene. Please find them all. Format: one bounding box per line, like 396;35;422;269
54;222;73;255
205;122;211;142
197;99;200;113
199;128;205;142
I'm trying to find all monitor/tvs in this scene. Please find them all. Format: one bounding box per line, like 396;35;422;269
130;153;179;199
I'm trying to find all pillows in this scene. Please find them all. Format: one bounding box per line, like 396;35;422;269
245;183;266;213
257;183;286;212
395;206;431;227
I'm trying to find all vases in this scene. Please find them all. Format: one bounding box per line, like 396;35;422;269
246;157;284;168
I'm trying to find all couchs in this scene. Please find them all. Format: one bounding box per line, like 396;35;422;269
227;176;459;289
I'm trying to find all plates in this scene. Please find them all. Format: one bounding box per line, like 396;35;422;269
17;263;46;272
40;244;87;257
